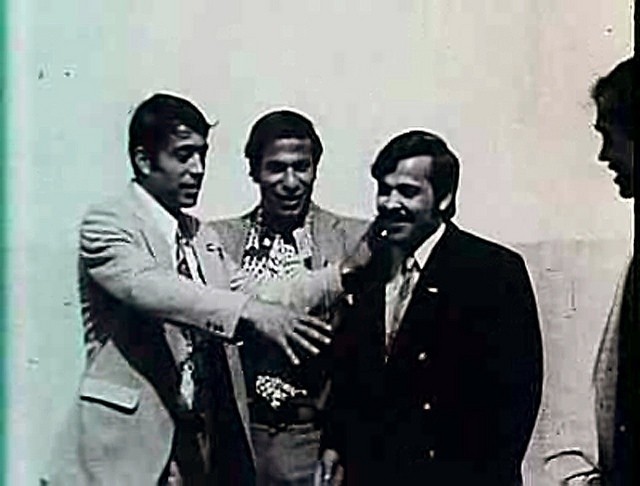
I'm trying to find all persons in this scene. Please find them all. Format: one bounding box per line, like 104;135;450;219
202;110;376;486
313;129;546;486
39;92;357;486
580;55;640;486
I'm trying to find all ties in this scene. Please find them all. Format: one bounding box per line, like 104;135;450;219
388;257;415;352
